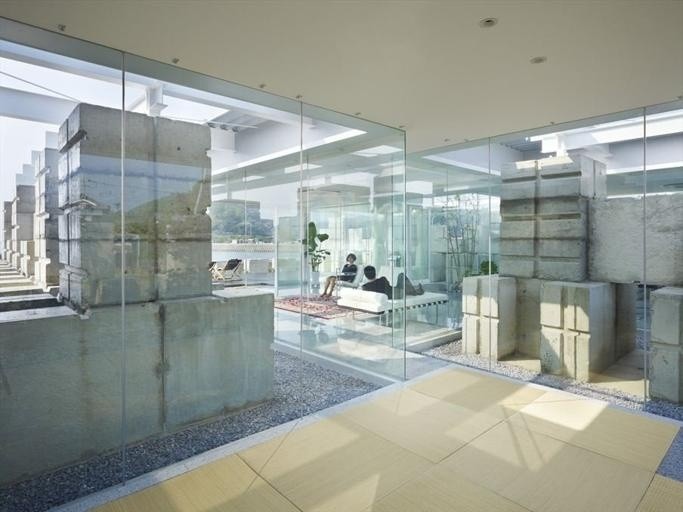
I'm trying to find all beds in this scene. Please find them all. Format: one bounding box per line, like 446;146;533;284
336;287;448;328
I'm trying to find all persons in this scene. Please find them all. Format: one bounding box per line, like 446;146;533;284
318;253;357;301
361;265;424;300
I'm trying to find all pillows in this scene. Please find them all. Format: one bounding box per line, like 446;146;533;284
338;287;387;306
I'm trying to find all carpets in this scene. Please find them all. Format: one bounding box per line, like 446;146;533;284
273;293;365;320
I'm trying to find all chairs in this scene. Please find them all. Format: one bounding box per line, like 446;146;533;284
322;264;363;299
208;259;241;282
